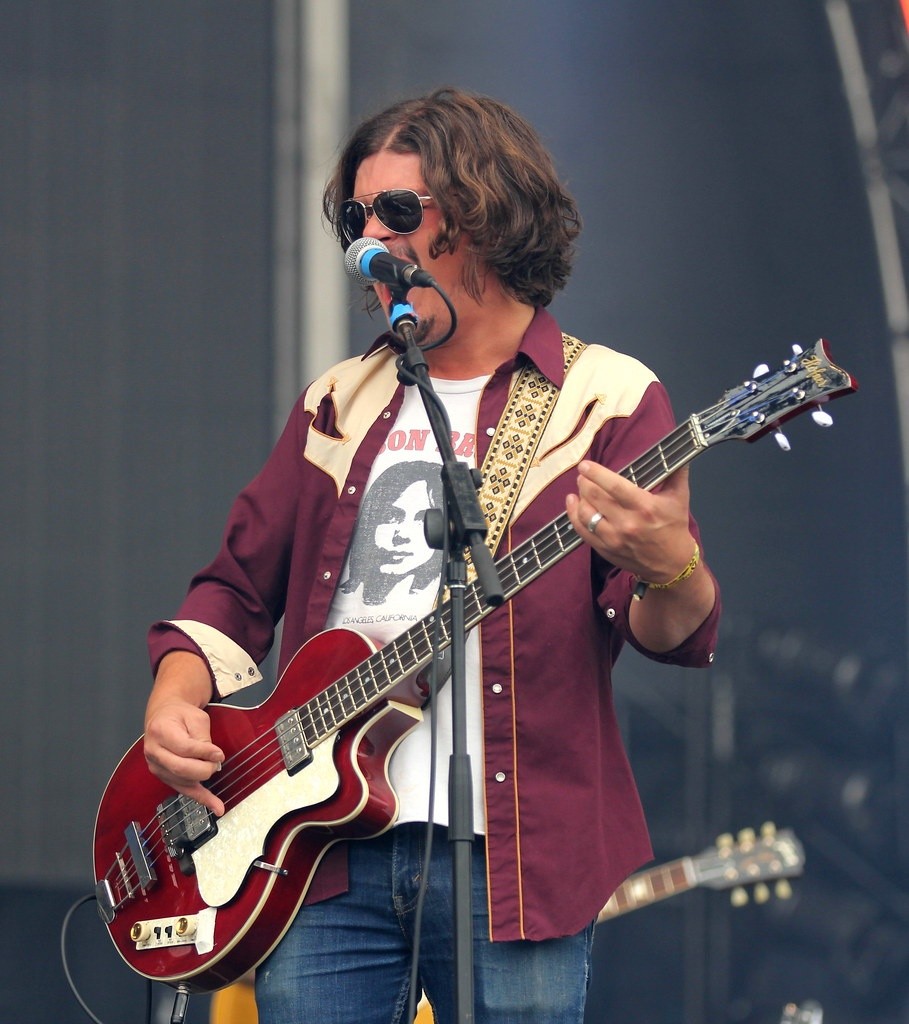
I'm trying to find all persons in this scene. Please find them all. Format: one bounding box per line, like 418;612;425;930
141;84;721;1024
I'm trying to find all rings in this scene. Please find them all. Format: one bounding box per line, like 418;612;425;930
586;511;603;532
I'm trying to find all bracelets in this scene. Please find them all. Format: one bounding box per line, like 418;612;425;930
633;532;699;602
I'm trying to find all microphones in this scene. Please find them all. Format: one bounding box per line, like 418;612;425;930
343;236;434;287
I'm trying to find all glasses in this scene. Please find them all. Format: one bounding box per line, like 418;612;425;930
337;188;436;246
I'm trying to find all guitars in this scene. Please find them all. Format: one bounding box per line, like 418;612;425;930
210;819;807;1024
89;328;859;994
